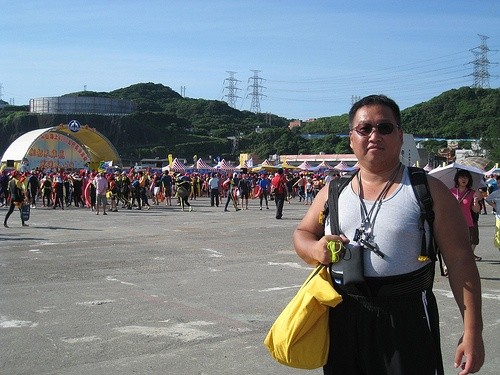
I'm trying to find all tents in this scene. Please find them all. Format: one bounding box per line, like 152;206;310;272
163;157;434;173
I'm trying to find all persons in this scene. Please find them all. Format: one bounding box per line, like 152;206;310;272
0;168;500;263
292;95;485;375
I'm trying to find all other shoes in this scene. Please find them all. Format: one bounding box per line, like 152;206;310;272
475;255;482;261
236;209;241;211
22;224;29;226
3;223;8;227
224;210;230;212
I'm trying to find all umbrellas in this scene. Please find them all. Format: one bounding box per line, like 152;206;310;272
428;161;487;192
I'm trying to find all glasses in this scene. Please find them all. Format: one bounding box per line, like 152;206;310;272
350;122;400;136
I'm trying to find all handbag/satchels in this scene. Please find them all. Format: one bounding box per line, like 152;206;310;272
20;200;30;221
274;188;279;194
264;179;343;370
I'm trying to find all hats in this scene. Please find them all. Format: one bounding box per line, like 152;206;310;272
12;170;22;176
114;171;126;174
